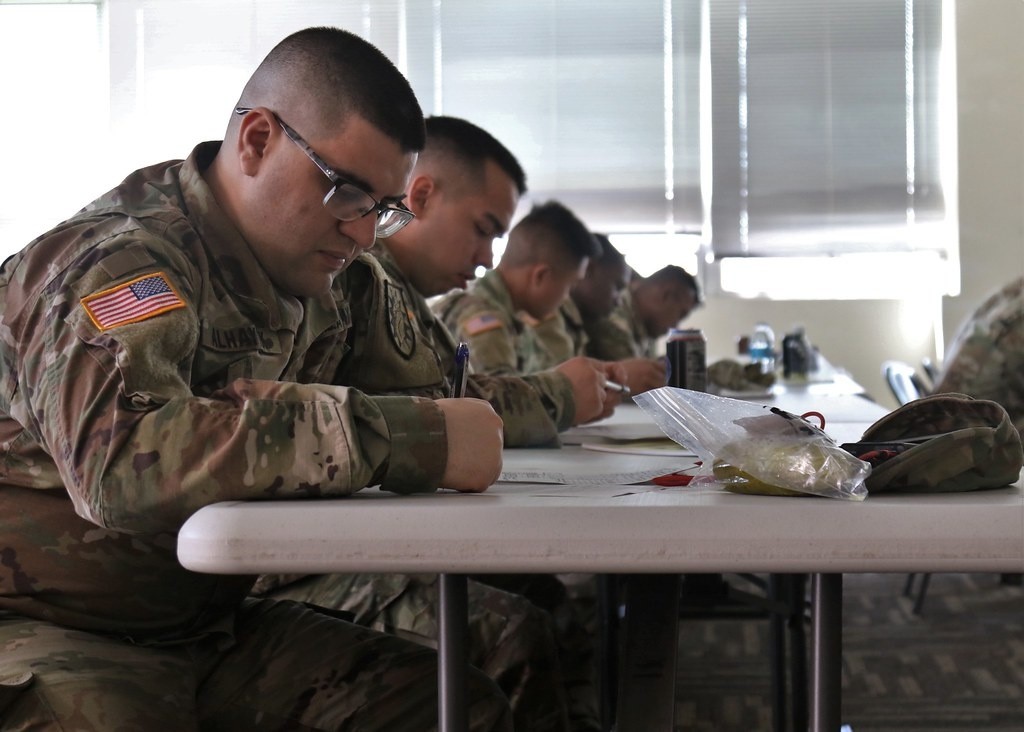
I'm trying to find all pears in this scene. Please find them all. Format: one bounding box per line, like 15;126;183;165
712;438;832;496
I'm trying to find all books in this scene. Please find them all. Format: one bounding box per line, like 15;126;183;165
580;436;704;458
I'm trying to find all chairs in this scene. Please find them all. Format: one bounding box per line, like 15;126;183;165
884;361;931;625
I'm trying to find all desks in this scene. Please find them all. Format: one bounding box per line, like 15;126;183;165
646;354;873;399
168;391;1024;731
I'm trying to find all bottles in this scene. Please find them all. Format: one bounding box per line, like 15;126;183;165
750;322;775;375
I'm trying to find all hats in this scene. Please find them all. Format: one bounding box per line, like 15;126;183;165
855;393;1023;492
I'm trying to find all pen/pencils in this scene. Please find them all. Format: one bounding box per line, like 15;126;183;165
450;341;471;400
602;380;631;397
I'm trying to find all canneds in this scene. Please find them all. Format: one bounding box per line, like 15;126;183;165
782;333;808;383
665;329;707;393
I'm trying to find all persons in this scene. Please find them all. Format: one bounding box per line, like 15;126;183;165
424;197;673;409
233;115;626;732
592;264;700;383
1;28;514;732
934;277;1024;587
517;230;625;383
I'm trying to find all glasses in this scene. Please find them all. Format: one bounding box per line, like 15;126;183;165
236;107;417;239
